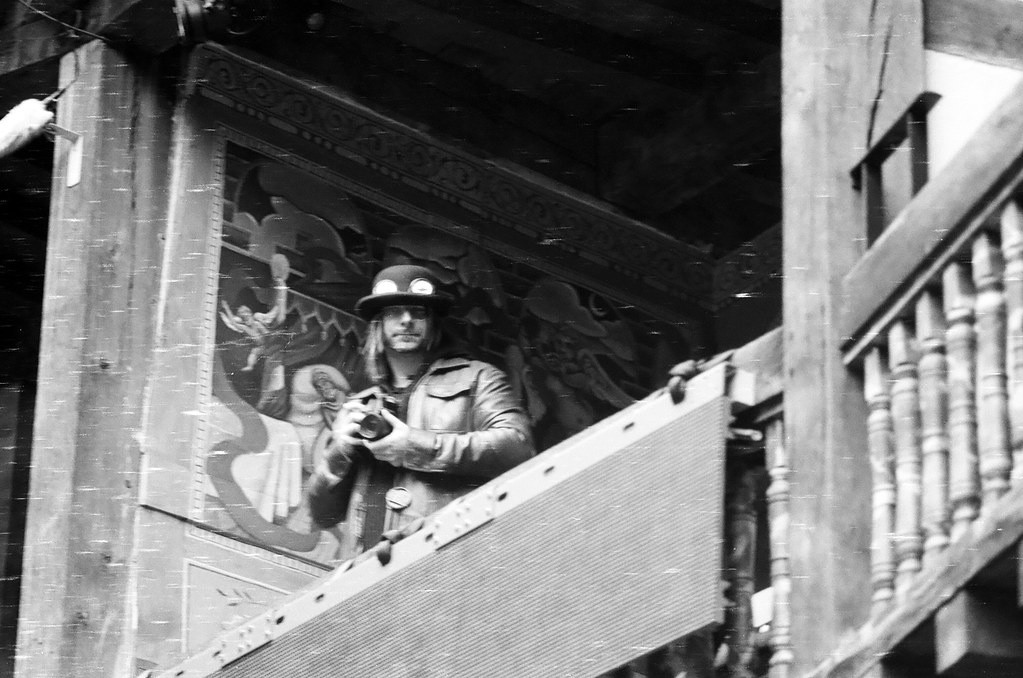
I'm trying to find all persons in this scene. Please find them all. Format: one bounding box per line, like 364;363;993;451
304;265;538;566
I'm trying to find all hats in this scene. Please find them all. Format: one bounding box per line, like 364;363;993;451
354;264;457;322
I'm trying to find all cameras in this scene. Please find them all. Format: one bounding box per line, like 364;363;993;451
351;393;399;442
